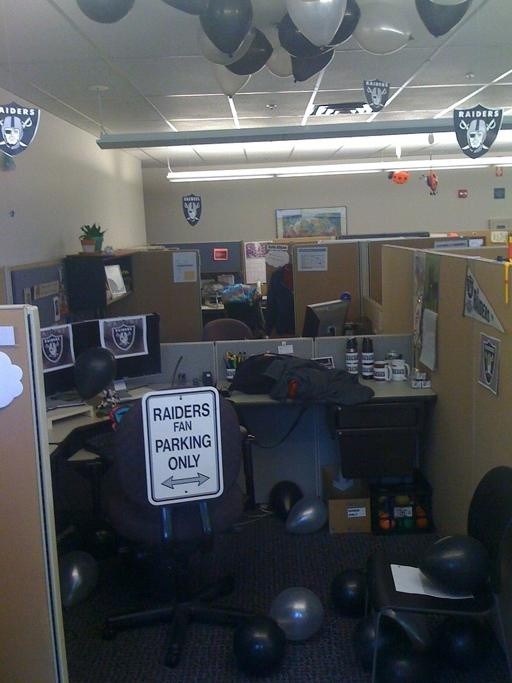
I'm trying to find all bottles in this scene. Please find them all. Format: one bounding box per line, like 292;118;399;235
345;338;359;377
360;336;375;379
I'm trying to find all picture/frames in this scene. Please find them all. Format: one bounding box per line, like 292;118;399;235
276;206;348;239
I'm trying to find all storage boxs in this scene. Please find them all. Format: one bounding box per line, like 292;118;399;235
323;460;374;536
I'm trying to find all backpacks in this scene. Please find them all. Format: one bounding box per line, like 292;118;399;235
230;352;375;411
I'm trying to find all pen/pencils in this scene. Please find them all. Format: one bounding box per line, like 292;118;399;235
224;351;247;369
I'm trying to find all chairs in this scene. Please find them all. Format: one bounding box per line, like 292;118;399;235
41;311;161;395
365;466;511;683
203;298;269;340
95;395;260;669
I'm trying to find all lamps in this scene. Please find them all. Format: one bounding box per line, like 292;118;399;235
90;82;511;184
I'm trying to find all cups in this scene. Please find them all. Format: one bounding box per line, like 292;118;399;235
389;359;410;381
373;360;393;382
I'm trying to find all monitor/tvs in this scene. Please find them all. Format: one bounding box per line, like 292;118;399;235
468;236;485;247
302;299;348;342
40;313;162;412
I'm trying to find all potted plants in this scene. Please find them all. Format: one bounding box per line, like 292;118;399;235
78;222;107;252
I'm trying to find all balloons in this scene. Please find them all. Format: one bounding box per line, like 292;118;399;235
58;551;97;616
267;480;302;520
231;534;491;683
73;347;116;400
284;495;328;541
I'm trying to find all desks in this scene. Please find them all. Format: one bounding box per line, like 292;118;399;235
45;362;443;507
